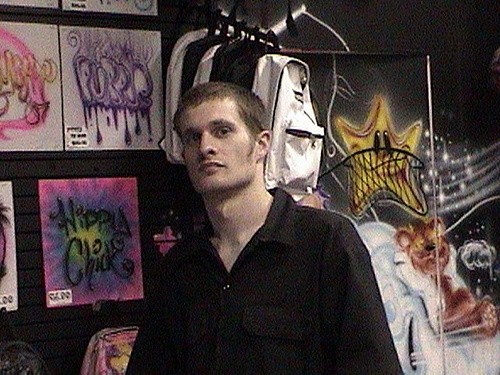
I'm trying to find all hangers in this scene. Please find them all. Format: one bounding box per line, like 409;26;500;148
196;6;286;62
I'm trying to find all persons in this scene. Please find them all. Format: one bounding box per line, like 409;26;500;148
124;82;404;375
